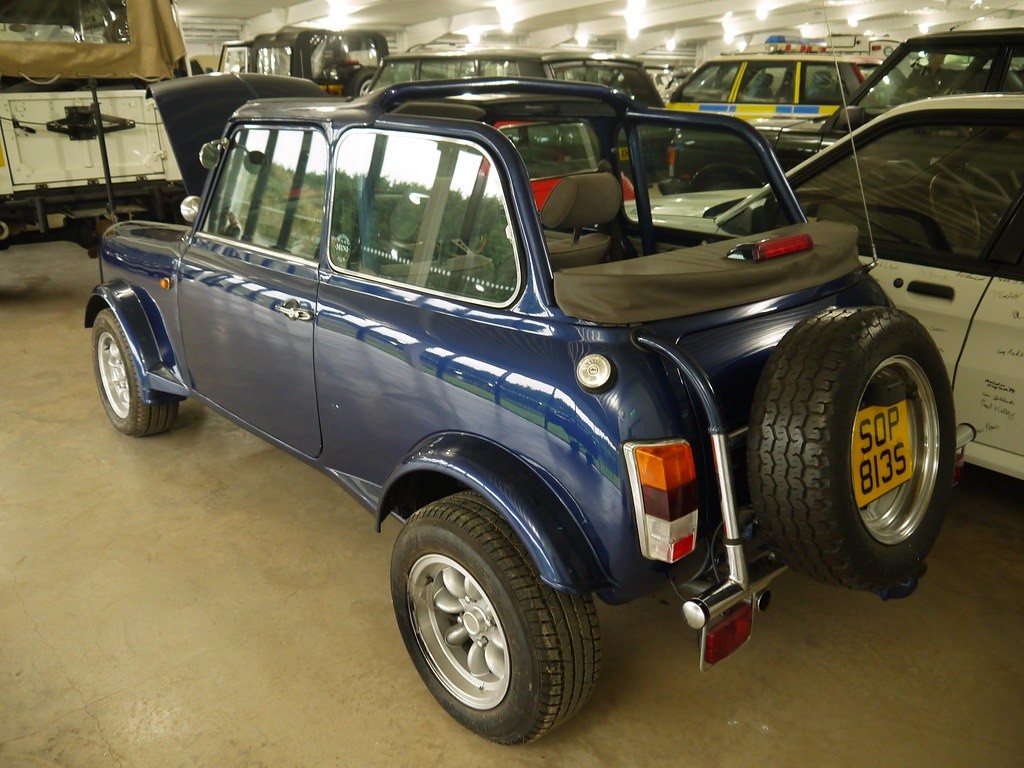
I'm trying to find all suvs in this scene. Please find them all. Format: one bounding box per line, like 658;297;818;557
660;26;1024;208
336;52;677;194
84;76;977;747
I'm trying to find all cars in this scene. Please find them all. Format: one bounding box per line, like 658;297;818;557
668;32;906;151
623;91;1024;485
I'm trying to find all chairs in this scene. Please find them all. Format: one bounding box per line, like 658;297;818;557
370;182;493;298
541;171;623;274
805;71;834;102
748;74;772;98
